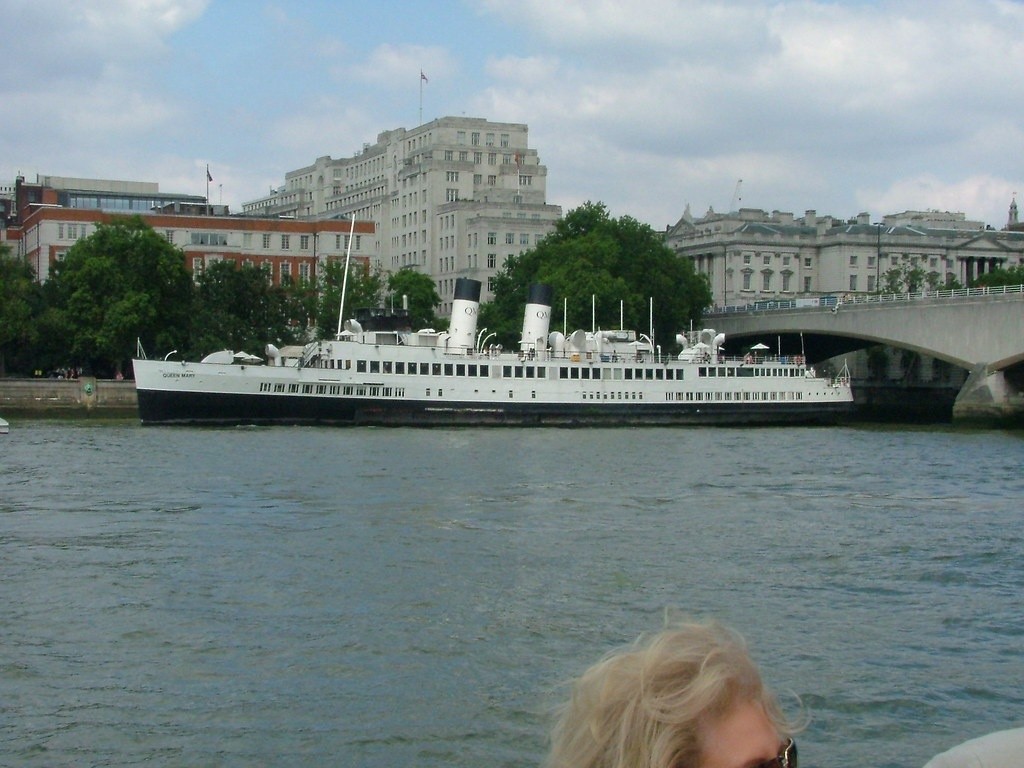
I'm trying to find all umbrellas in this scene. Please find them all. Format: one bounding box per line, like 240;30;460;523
246;354;260;365
750;343;770;350
234;351;249;365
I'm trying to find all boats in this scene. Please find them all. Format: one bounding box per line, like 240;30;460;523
132;212;862;432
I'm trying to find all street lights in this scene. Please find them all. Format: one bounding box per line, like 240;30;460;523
872;222;885;295
717;241;729;307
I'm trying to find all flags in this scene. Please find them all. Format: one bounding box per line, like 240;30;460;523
422;73;428;83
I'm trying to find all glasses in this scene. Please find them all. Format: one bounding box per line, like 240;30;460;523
757;737;798;768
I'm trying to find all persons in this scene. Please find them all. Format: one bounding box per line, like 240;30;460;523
548;604;812;768
743;352;753;365
54;367;80;379
779;355;802;365
